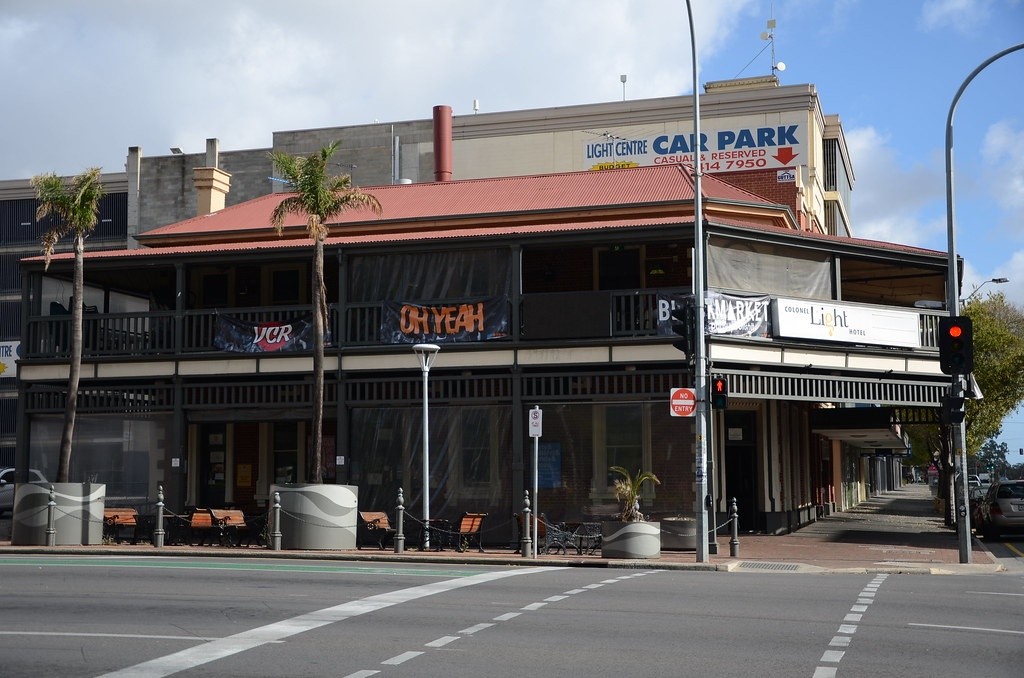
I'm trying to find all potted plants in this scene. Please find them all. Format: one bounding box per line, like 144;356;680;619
602;466;661;559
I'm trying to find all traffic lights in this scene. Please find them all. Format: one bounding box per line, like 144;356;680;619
673;297;696;363
712;377;730;410
939;318;974;373
943;394;966;425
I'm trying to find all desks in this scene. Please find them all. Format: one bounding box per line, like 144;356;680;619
556;521;602;554
134;514;188;543
417;519;456;549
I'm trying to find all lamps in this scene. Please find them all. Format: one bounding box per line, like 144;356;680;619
170;147;185;155
621;74;627;100
915;299;949;308
960;277;1010;298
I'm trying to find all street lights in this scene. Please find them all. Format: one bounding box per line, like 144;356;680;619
913;276;1009;565
412;342;441;550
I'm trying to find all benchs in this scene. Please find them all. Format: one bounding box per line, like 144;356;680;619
358;511;395;551
210;508;251;548
435;511;490;552
172;512;211;547
102;508;139;545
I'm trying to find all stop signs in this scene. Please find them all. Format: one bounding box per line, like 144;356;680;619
670;388;698;416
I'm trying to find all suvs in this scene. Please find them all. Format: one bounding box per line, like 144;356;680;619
979;481;1024;541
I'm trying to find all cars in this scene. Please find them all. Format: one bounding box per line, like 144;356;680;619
956;473;1014;521
1;468;50;515
80;471;147;501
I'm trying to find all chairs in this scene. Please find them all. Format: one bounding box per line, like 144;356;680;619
513;512;549;555
50;296;99;351
